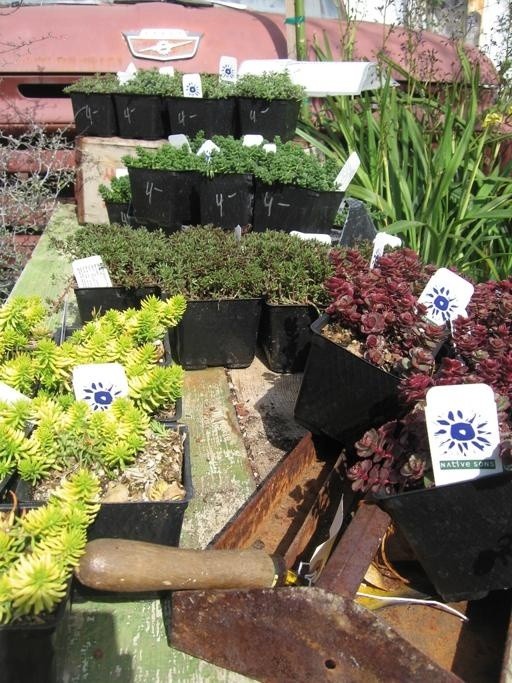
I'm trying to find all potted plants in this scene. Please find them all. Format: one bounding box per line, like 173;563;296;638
63;76;115;138
0;426;193;507
0;362;187;422
124;141;198;233
117;71;171;140
164;73;236;140
0;296;185;371
195;134;263;234
0;470;97;630
169;228;264;368
255;135;347;240
6;293;184;365
254;230;336;372
236;79;303;141
103;175;137;232
60;223;176;366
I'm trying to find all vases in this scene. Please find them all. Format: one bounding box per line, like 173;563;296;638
384;472;512;608
296;308;415;452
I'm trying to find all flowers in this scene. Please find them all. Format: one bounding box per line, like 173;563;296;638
329;248;449;362
346;371;512;492
449;279;512;390
